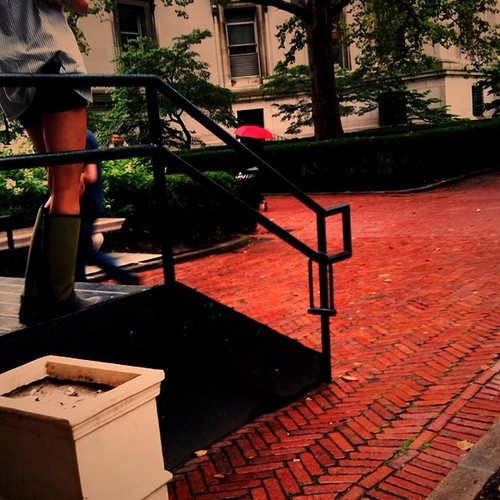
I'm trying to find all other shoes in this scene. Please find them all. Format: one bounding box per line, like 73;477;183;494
122;275;140;286
261;200;268;212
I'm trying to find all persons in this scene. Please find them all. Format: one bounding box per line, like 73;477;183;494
0;1;104;331
240;134;271;212
44;123;146;289
106;131;128;148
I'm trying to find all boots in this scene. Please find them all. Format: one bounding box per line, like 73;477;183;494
29;210;101;320
17;200;49;326
